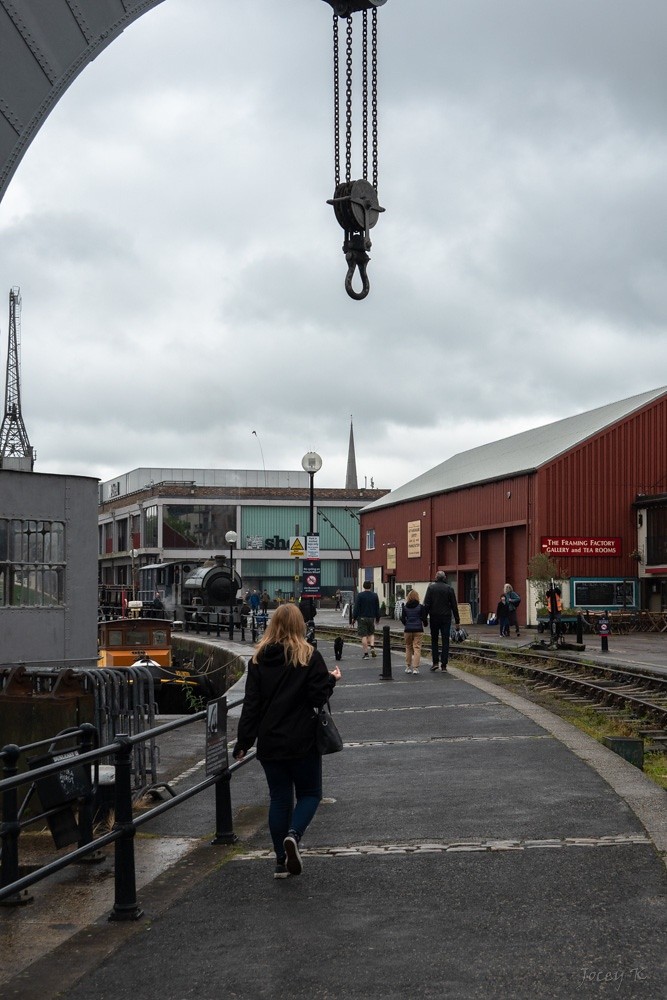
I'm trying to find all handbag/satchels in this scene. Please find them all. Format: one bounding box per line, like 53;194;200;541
314;690;344;756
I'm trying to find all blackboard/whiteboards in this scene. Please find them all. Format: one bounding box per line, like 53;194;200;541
456;603;474;625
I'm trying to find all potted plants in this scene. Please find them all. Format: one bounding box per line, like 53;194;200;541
527;552;579;619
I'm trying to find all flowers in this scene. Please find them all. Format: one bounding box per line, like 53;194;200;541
629;549;642;559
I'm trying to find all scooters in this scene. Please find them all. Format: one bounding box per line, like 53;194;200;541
304;621;315;641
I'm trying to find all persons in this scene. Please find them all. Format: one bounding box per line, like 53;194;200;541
423;571;460;673
334;588;342;612
352;580;380;660
401;589;428;674
232;603;342;878
495;584;520;637
298;597;314;624
243;587;271;616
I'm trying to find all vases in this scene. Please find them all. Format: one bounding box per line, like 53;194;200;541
634;556;644;566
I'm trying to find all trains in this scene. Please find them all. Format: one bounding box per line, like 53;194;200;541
98;554;243;631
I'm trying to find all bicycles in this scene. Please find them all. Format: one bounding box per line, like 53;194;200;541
247;620;265;642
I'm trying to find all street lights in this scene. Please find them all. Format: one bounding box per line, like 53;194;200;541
302;451;323;646
317;508;356;604
225;530;238;641
129;548;137;601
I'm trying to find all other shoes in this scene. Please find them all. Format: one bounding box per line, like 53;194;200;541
516;632;520;637
361;648;378;659
499;632;510;638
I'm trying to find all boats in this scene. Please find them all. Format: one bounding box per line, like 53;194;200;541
99;615;201;712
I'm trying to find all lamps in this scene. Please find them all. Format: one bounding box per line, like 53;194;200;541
360;492;363;497
344;506;360;525
191;490;195;494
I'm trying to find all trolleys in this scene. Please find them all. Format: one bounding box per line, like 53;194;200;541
535;600;578;632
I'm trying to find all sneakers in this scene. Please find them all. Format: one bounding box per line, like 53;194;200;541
274;835;304;879
404;666;421;675
430;664;448;673
